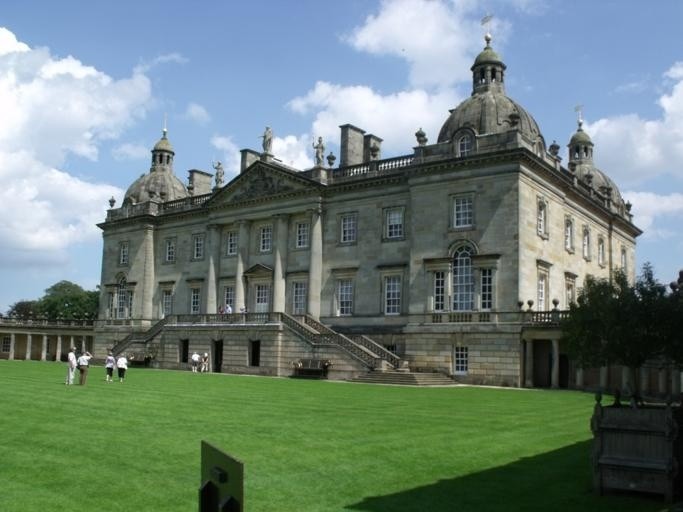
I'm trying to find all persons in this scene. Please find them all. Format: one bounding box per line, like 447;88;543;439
104;351;128;383
212;160;225;186
312;136;325;165
262;126;273;152
191;351;209;373
217;303;233;319
65;346;93;385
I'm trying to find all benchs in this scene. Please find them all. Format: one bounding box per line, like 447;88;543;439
286;358;332;380
125;350;152;367
